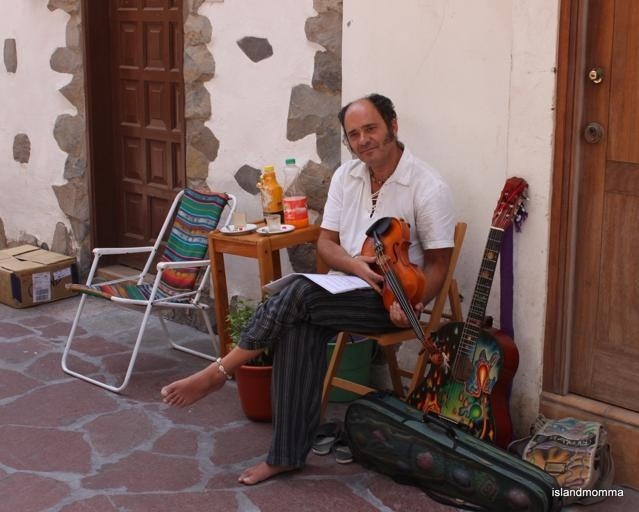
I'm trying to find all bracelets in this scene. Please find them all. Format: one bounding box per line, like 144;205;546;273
215;356;234;380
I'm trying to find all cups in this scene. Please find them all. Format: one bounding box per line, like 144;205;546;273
265;213;282;231
232;213;247;231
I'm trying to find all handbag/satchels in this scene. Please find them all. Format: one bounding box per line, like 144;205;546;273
507;412;617;504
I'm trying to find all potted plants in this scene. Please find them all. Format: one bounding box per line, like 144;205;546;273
223;297;275;419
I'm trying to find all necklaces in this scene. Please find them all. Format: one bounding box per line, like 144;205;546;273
368;167;391;186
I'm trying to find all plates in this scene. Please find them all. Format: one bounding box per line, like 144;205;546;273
219;223;258;235
256;224;296;234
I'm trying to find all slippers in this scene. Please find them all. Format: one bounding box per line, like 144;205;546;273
334;437;355;465
309;416;340;456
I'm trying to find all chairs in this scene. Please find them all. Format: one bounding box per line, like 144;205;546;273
61;187;238;393
320;222;468;428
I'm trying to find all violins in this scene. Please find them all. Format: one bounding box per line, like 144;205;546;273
360;216;451;378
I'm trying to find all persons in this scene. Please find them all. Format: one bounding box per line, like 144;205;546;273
160;92;456;486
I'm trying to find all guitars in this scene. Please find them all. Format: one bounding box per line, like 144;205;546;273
403;175;530;455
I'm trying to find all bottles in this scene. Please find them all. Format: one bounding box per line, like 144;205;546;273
261;165;283;222
285;158;308;227
